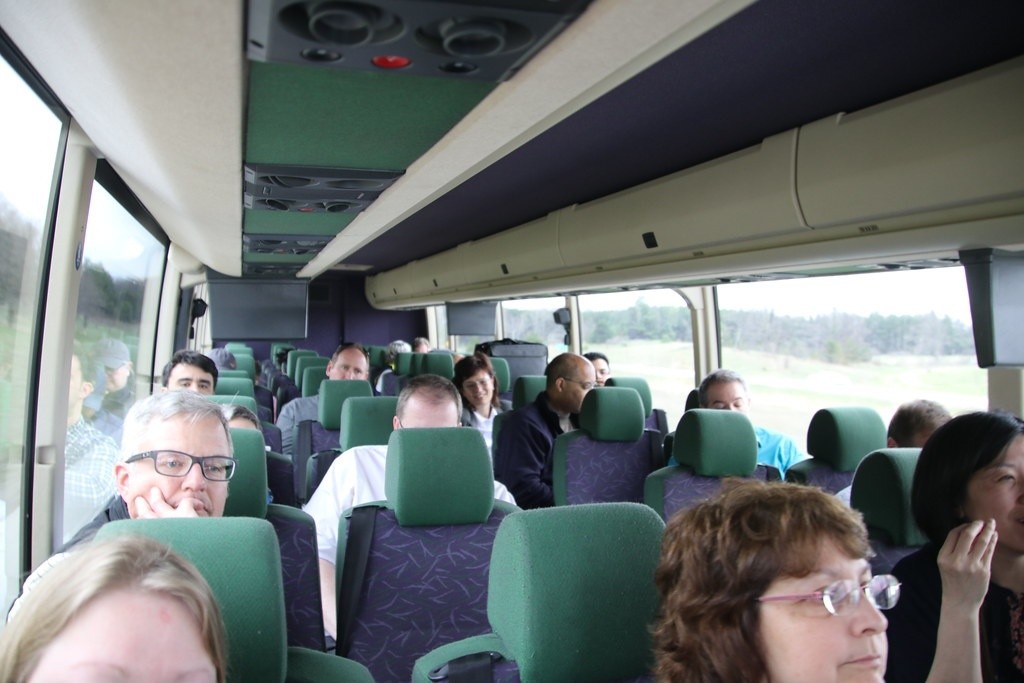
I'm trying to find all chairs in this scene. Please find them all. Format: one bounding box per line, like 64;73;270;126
93;341;928;682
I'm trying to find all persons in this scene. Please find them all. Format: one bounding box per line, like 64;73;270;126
494;353;596;510
161;350;218;394
649;480;901;683
668;371;803;480
53;391;234;553
220;404;273;504
303;374;518;643
0;537;230;683
63;339;136;545
275;342;371;457
584;352;610;387
374;339;411;396
835;401;951;508
452;351;513;463
876;413;1023;683
412;337;430;352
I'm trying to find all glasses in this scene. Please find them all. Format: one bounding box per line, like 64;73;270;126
338;342;370;356
565;377;596;390
760;573;904;615
126;450;239;483
